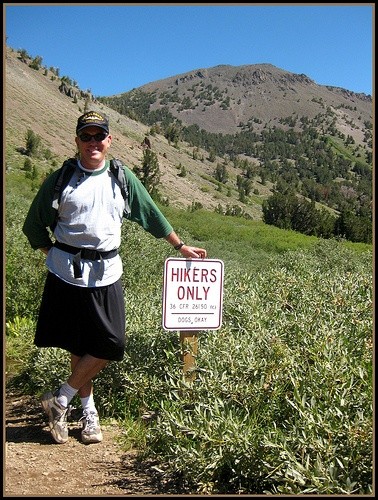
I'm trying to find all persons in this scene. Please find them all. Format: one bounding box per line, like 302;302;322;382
22;112;206;444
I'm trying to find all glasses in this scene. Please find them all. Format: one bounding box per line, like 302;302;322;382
79;132;109;142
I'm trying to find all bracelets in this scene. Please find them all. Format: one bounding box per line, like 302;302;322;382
173;239;185;250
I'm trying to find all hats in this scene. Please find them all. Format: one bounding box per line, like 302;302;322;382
77;111;110;132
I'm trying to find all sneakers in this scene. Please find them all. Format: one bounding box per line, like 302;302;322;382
42;396;76;444
77;409;103;444
